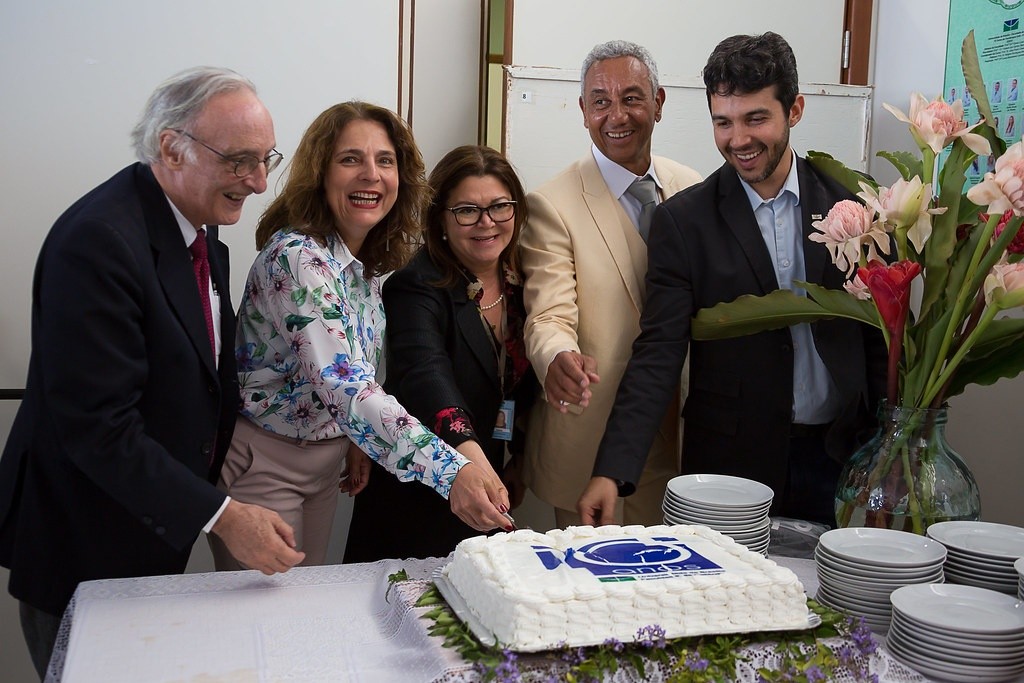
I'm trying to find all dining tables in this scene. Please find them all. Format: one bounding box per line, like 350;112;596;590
41;554;948;683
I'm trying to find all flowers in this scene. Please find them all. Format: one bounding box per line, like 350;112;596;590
691;28;1024;538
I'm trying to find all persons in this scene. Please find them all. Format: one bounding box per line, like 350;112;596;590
944;74;1024;178
1;66;308;683
339;144;541;565
511;39;708;534
577;31;918;531
495;412;507;430
198;98;517;577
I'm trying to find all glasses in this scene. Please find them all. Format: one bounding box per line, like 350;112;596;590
178;128;284;179
442;200;518;227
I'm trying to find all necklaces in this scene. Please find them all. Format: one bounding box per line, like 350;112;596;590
481;294;504;311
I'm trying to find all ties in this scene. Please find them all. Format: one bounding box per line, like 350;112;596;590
191;231;216;371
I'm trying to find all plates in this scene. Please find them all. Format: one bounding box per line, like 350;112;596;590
661;473;773;558
814;521;1024;683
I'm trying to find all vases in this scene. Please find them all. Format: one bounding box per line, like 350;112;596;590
832;396;985;539
